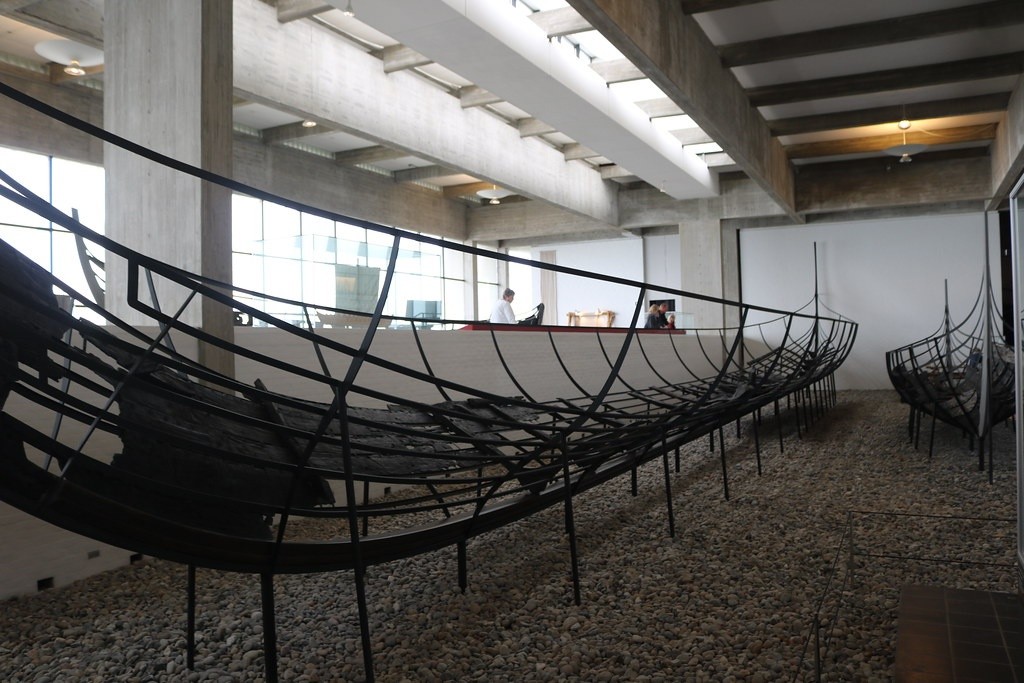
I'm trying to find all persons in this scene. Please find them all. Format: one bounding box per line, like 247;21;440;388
645;303;676;329
489;288;518;324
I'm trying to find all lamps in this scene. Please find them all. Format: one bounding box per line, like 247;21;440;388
34;39;105;76
302;118;317;128
475;184;512;205
883;102;929;163
